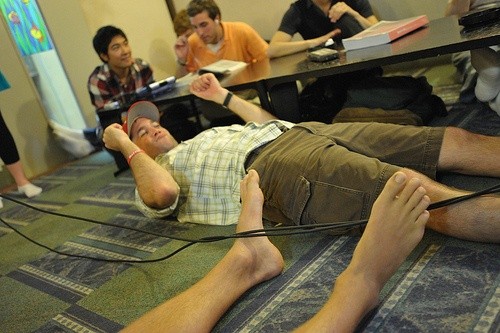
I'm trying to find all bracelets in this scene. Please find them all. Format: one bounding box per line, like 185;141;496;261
223;92;234;110
126;149;146;165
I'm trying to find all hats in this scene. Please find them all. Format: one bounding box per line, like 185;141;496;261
121;101;161;137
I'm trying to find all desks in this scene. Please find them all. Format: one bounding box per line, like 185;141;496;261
96;14;500;178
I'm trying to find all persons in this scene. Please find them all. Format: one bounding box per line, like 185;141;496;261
270;0;382;78
0;71;42;208
120;169;431;333
445;0;500;116
103;74;500;244
49;0;269;159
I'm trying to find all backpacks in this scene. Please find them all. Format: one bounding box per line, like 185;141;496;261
297;74;449;127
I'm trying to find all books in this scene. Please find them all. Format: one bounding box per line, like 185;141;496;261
342;15;430;50
175;60;247;86
